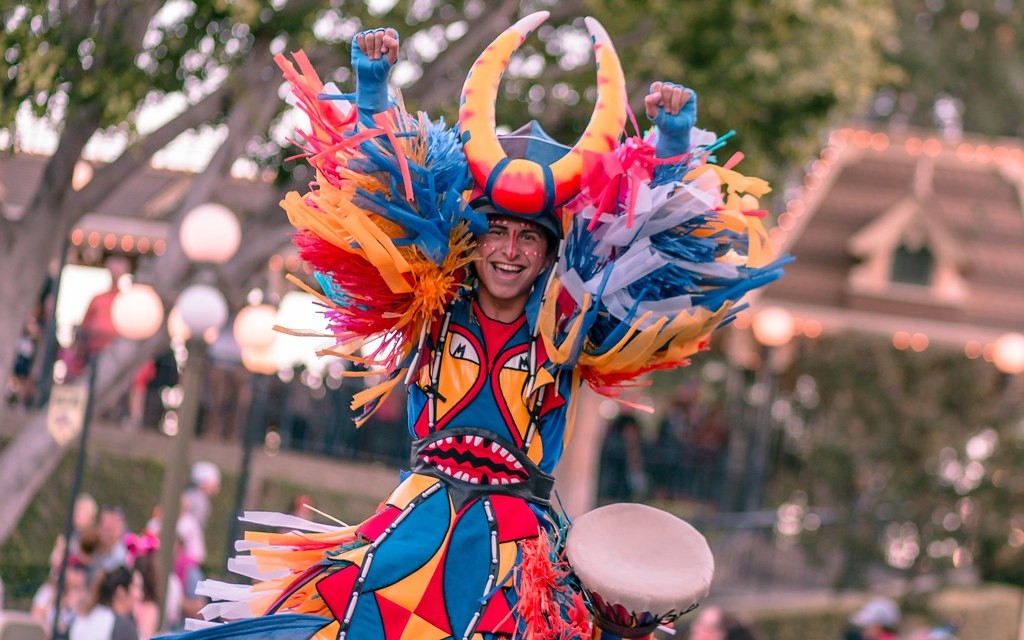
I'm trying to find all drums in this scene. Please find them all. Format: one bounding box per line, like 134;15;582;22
562;499;717;640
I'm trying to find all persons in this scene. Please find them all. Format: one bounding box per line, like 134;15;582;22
29;456;220;640
311;25;699;640
281;493;318;542
677;603;748;640
847;595;906;639
